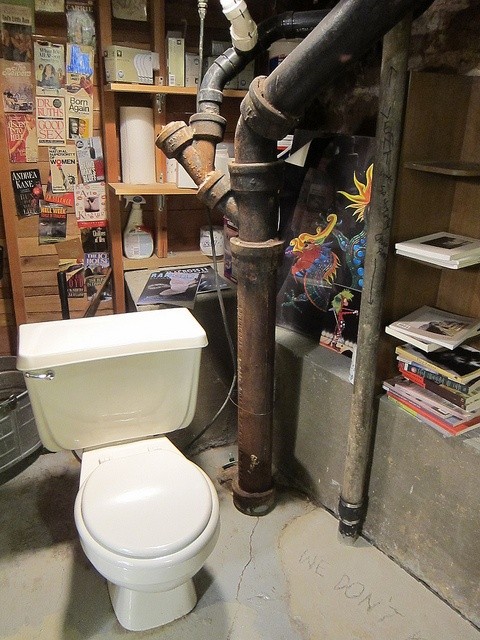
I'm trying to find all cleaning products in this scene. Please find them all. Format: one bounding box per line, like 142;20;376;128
122;194;154;259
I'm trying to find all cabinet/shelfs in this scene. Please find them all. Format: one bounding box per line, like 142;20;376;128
377;52;479;388
97;0;249;315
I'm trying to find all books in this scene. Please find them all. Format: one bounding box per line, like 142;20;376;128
161;264;230;293
381;304;480;439
137;269;202;310
394;232;480;271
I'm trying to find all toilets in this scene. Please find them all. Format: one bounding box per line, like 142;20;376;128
16;307;220;632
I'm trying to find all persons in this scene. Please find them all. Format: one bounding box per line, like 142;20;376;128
57;69;63;87
35;64;44;84
0;24;33;62
76;119;85;137
39;203;66;238
9;115;38;162
42;64;61;87
58;163;76;191
70;118;78;137
